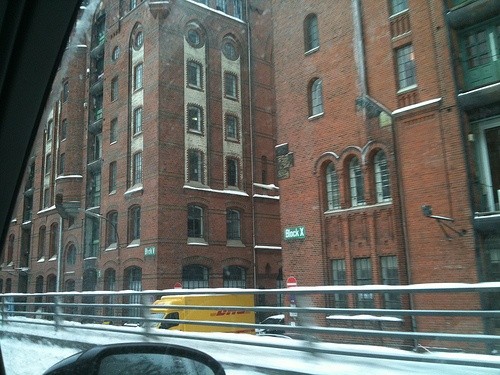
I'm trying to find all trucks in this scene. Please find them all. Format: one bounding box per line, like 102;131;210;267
145;294;255;336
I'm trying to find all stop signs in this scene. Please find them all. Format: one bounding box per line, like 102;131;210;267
287;276;297;288
173;282;183;289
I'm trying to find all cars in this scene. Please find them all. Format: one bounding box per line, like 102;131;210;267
255;314;297;335
